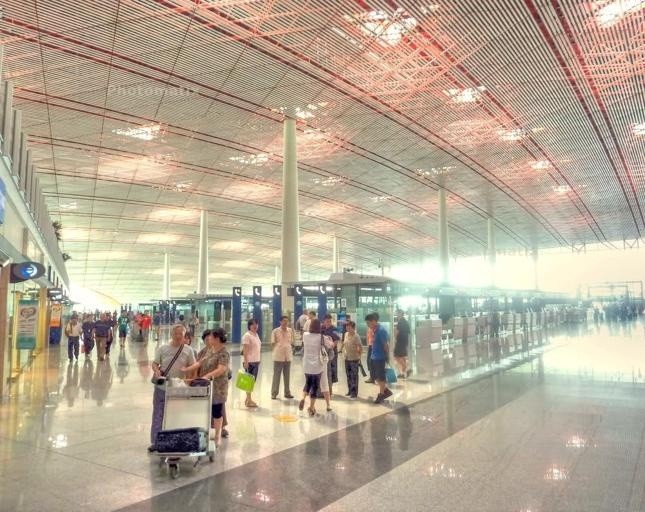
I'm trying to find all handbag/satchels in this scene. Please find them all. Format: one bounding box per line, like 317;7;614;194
151;364;163;385
384;363;397;382
235;367;256;392
319;345;329;367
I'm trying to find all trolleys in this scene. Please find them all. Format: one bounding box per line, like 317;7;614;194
146;377;216;478
291;330;304;359
130;320;142;342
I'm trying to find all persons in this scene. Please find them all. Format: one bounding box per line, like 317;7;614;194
338;315;350;353
344;321;363;397
66;308;160;360
440;298;644;336
148;325;229;452
364;309;410;401
299;311;342;412
240;318;262;406
270;316;293;397
